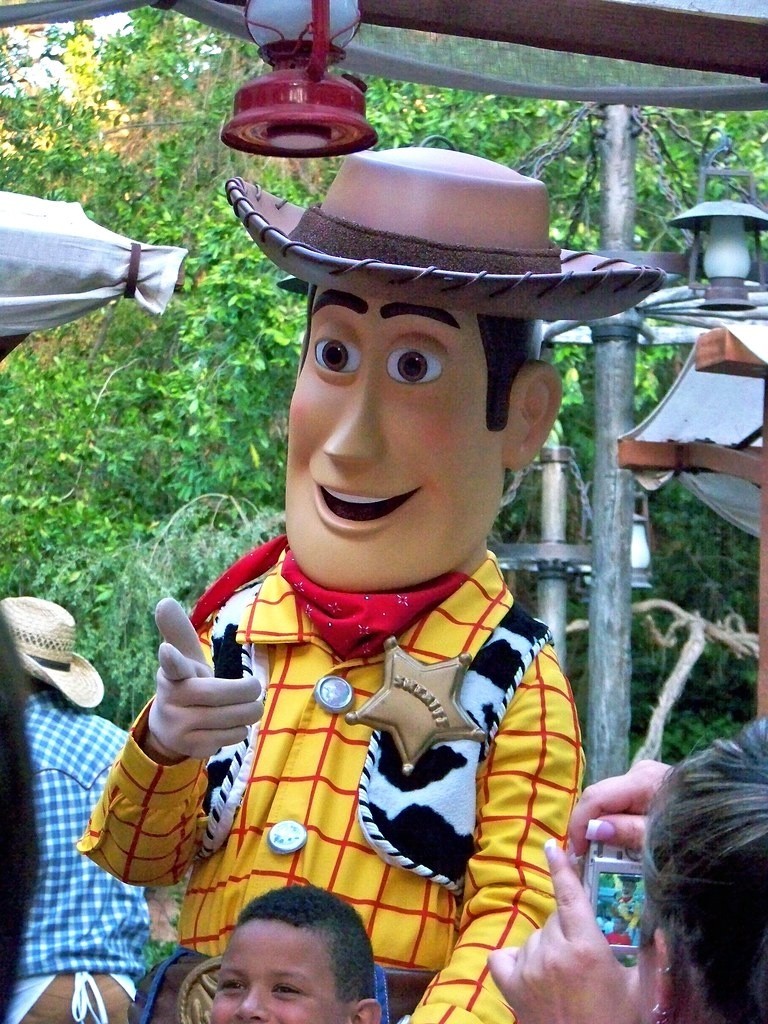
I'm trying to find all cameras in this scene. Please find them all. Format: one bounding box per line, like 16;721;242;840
582;840;645;954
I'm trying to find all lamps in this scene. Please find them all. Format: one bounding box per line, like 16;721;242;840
632;491;656;591
666;126;768;314
220;0;381;159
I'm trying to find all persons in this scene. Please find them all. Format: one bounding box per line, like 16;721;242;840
0;595;151;1024
488;715;767;1024
615;873;644;946
596;914;631;946
79;144;668;1024
210;883;382;1024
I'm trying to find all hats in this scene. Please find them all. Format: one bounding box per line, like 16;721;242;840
0;595;107;709
